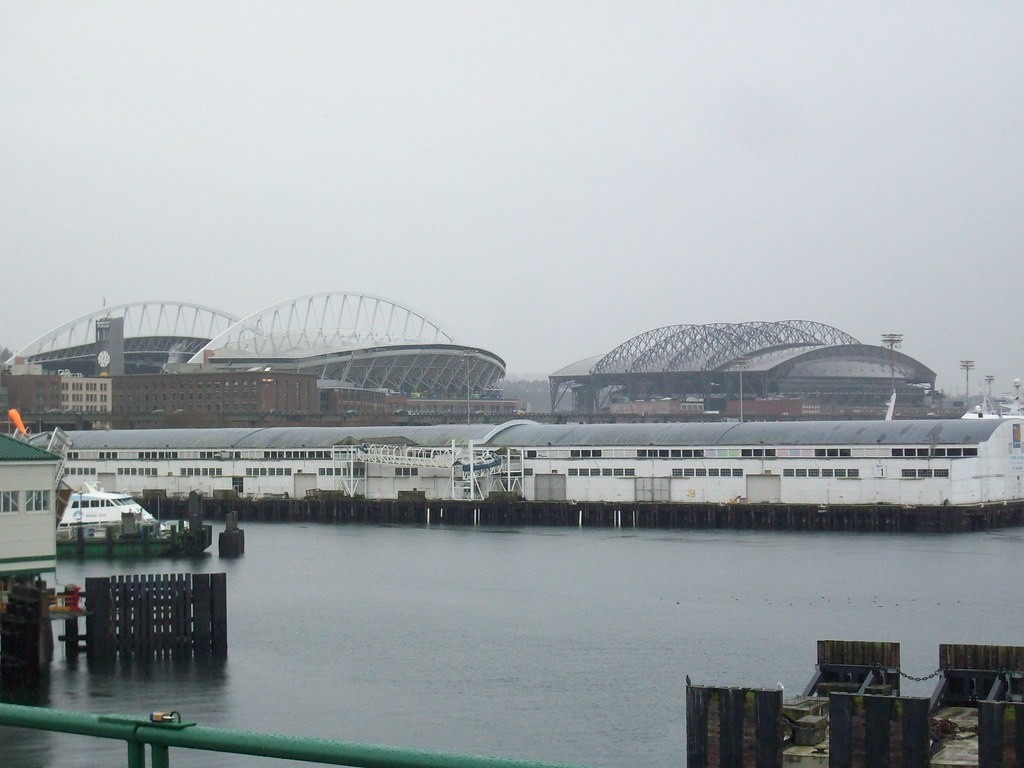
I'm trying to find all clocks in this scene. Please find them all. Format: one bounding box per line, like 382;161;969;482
97;350;111;368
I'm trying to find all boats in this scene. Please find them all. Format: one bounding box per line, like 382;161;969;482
55;482;164;537
